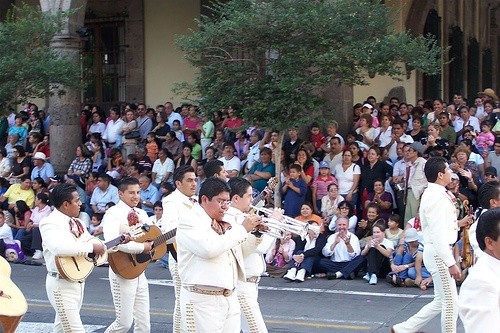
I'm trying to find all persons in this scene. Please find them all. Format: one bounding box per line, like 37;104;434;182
0;88;500;290
221;176;285;333
391;157;476;333
468;180;500;258
175;175;262;333
103;177;154;333
458;207;500;333
161;166;199;333
38;183;106;333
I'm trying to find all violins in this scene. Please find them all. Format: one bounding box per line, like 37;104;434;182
463;198;477;268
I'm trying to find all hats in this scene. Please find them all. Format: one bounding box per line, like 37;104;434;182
451;173;460;179
477;88;498;100
318;162;330;169
407;141;424;155
361;103;372;109
404;228;419;243
483;167;497;177
32;152;47;162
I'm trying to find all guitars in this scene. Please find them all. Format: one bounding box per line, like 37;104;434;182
244;176;280;213
167;243;177;263
0;255;29;333
107;224;177;281
54;223;151;285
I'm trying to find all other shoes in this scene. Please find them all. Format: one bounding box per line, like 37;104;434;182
405;278;417;286
327;272;335;279
350;272;354;279
369;275;378;285
363;274;370;281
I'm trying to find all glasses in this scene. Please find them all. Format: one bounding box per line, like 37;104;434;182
38;172;41;177
208;197;233;206
340;206;350;211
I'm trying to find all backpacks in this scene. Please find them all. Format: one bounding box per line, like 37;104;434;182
6;243;24;263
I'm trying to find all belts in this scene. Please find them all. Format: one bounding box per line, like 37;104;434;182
248;276;261;283
46;271;67;279
184;285;235;297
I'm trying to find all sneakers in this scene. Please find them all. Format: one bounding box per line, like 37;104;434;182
295;271;304;282
31;250;43;260
283;271;296;281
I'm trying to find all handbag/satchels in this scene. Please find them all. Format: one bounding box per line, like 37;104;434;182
124;119;140;138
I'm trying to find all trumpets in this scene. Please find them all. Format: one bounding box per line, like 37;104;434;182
223;211;307;241
248;204;323;239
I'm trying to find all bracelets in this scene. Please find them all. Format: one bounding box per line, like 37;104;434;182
186;128;188;130
